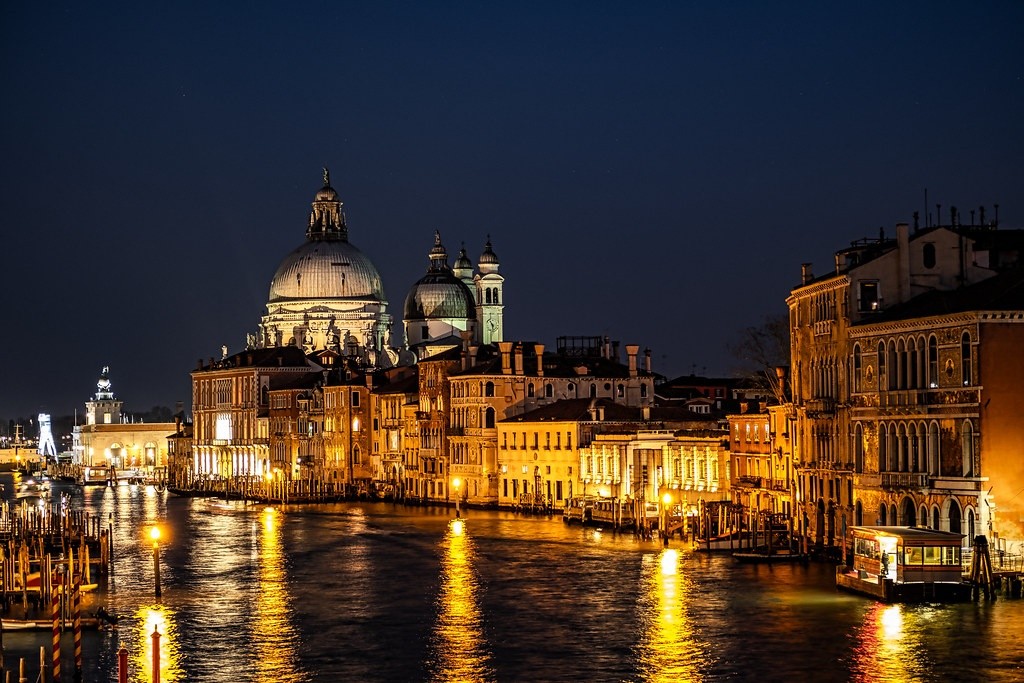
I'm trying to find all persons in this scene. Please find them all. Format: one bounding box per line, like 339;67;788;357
867;367;872;382
881;553;889;575
947;360;953;377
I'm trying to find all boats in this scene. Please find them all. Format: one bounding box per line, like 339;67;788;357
1;614;98;630
84;466;112;485
563;495;634;526
732;551;808;561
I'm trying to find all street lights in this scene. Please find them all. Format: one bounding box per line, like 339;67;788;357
15;455;20;470
104;448;111;465
120;448;127;470
88;447;94;466
